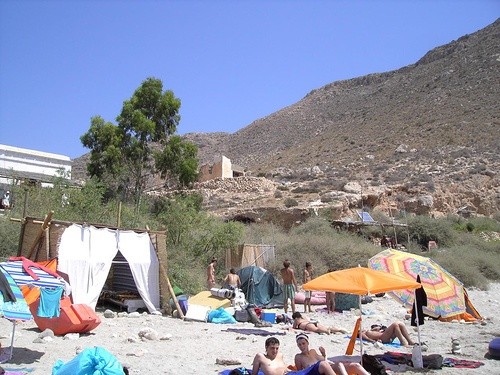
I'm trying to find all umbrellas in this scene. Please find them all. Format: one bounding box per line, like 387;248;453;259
345;318;361;355
301;263;421;357
367;248;466;321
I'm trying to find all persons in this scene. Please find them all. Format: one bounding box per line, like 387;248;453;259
207;257;417;375
380;236;397;249
2;190;10;210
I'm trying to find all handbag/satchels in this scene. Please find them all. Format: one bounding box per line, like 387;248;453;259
360;354;387;375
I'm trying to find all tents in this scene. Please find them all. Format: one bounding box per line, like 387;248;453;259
407;286;481;322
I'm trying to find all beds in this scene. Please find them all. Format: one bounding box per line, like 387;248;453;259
101;294;147;311
